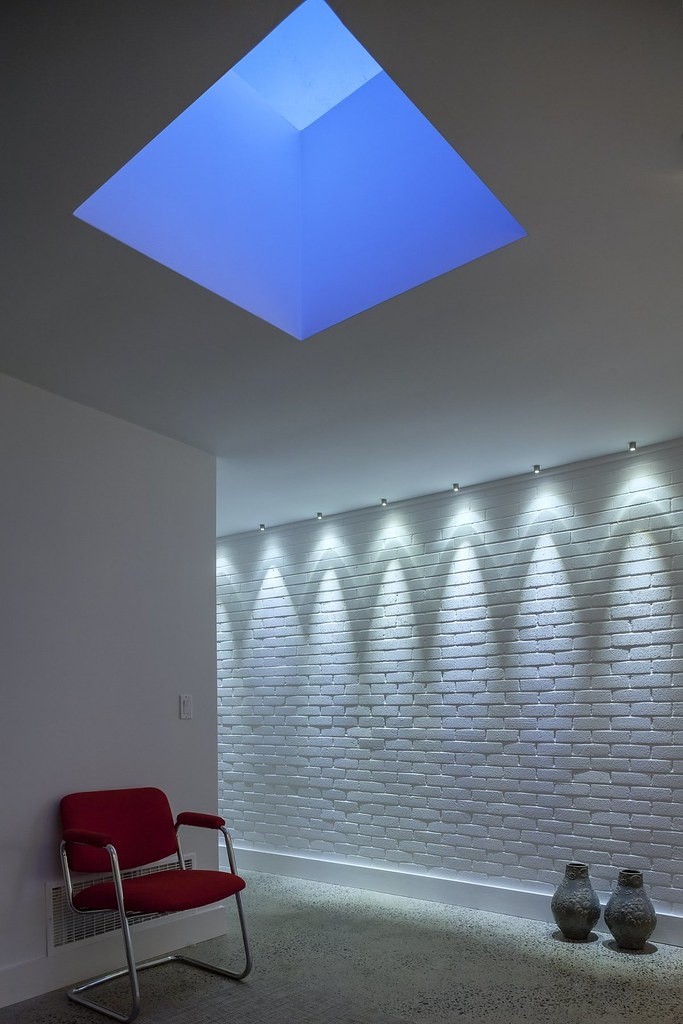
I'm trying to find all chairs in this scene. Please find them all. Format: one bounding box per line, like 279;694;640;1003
59;787;252;1023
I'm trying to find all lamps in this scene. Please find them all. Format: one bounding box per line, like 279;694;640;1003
260;524;264;531
382;499;386;506
629;442;636;451
534;465;540;473
317;513;322;519
453;484;459;491
